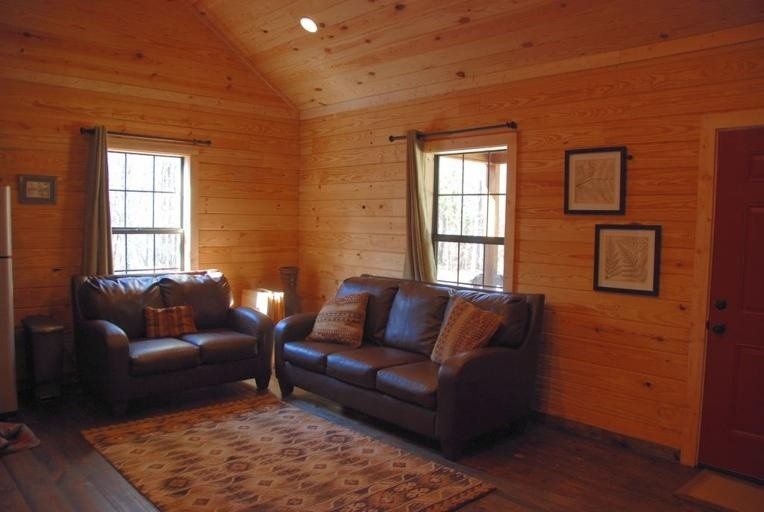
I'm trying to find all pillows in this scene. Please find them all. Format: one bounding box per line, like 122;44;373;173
306;294;372;348
147;302;197;339
429;296;505;367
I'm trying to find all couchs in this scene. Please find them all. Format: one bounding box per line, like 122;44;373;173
67;267;274;412
274;273;545;460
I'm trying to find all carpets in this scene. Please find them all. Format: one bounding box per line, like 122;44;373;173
81;393;496;510
673;467;764;512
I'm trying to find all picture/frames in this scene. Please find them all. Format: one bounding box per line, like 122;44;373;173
19;174;56;204
564;145;660;298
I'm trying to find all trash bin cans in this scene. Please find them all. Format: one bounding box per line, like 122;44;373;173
22;315;66;400
